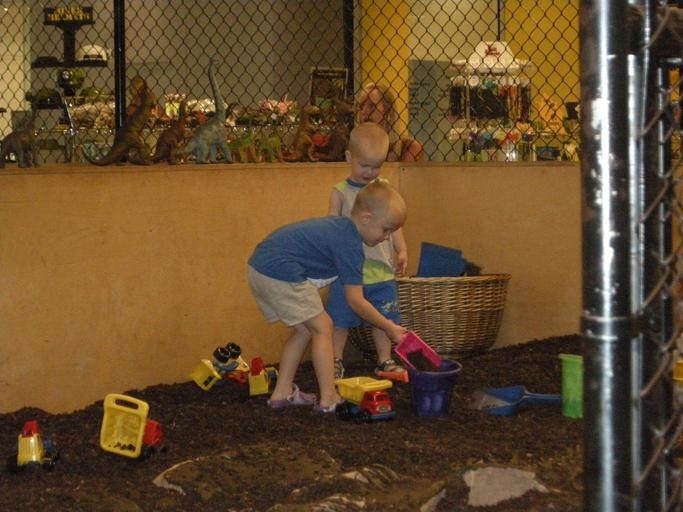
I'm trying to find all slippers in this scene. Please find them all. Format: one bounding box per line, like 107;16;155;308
268;384;344;413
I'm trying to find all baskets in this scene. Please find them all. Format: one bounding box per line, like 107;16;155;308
348;274;512;360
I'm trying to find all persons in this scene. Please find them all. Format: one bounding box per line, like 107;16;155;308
358;83;422;163
244;178;410;413
318;121;411;386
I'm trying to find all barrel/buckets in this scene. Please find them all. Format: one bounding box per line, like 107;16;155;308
559;353;584;419
407;358;462;415
